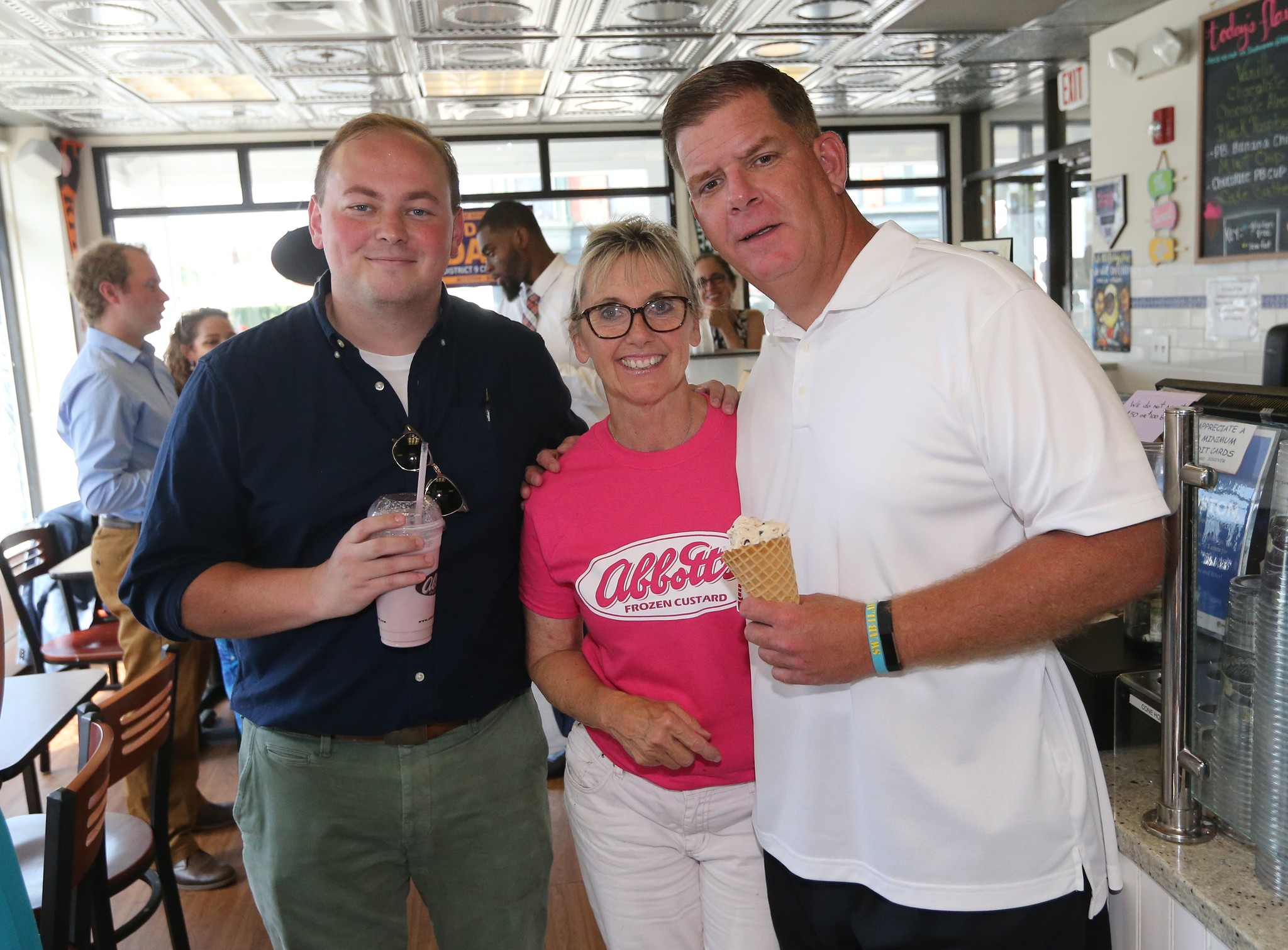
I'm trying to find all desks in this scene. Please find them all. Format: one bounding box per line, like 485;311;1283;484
0;667;107;813
51;541;241;747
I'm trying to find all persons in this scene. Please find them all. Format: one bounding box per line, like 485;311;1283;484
465;198;615;785
120;112;747;950
163;306;238;395
53;242;242;891
518;219;778;950
512;63;1173;950
693;253;764;349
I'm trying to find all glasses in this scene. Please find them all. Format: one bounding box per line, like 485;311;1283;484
571;295;692;340
392;423;470;517
696;272;728;288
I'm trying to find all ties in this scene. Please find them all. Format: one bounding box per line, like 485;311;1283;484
522;289;541;332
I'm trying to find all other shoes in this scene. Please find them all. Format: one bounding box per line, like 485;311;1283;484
547;748;567;778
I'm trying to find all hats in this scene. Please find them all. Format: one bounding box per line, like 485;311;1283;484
271;225;330;286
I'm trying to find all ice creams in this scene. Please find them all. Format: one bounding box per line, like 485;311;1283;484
720;514;800;603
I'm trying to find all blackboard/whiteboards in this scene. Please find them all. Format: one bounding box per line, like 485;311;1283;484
1193;1;1287;262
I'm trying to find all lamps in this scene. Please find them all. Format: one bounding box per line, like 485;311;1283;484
1106;24;1190;78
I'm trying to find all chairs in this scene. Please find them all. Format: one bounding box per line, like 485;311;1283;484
37;711;116;950
6;644;191;950
0;521;124;772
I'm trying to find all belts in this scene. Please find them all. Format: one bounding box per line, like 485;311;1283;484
311;719;479;747
97;515;139;528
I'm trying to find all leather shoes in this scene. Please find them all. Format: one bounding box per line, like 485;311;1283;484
173;849;238;891
191;801;235;833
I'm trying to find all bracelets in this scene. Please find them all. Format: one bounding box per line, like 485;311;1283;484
865;600;890;675
876;598;901;673
